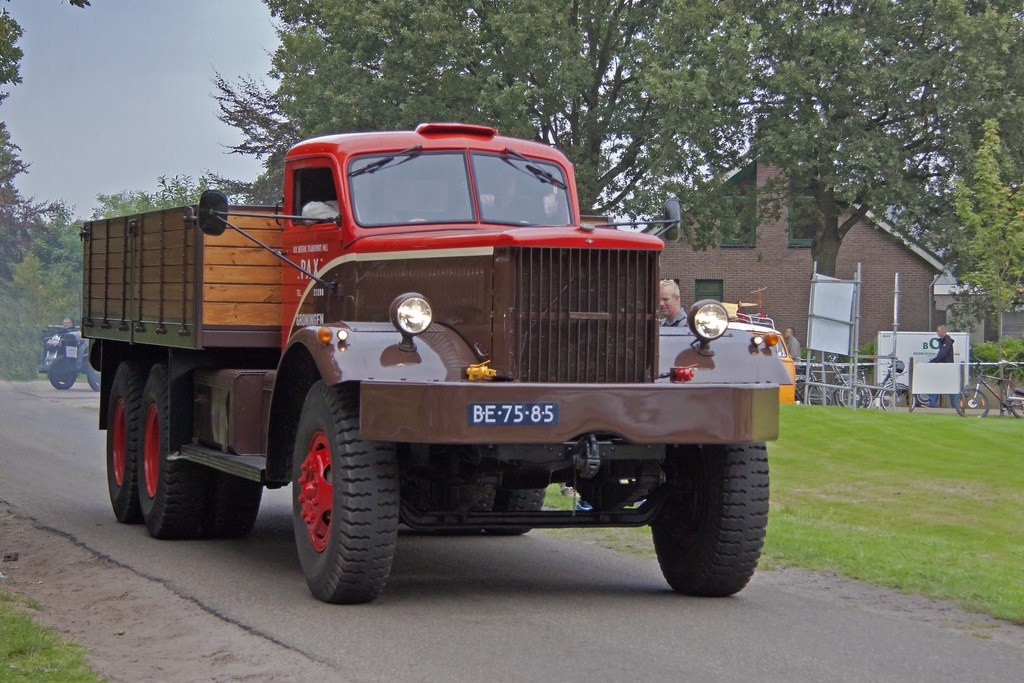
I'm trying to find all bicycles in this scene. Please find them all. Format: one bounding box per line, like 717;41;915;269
793;353;916;412
955;358;1024;419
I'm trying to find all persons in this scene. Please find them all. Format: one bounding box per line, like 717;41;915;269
784;327;802;376
660;279;689;327
302;161;367;225
60;318;79;338
919;325;958;409
478;168;557;216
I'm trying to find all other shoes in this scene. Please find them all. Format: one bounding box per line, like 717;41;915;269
561;486;582;498
576;501;593;511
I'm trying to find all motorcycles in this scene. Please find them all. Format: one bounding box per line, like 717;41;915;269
40;325;101;391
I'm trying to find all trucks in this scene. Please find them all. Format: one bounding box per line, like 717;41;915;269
78;122;792;606
